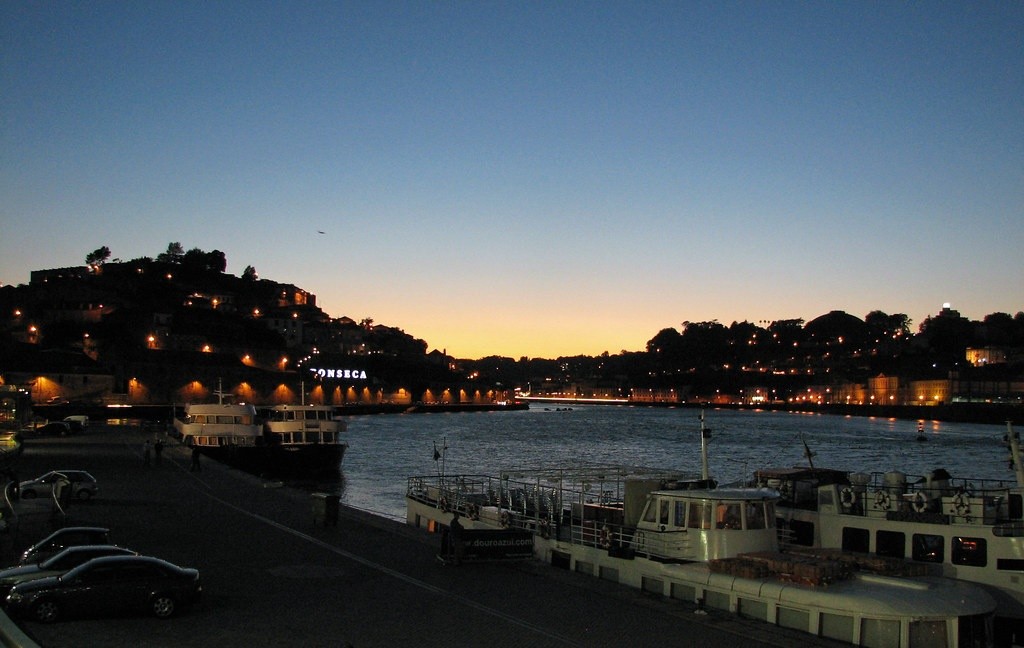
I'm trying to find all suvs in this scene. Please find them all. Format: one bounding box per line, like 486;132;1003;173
47;395;61;404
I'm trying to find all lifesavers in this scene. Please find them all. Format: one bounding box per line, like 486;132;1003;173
840;488;855;507
911;492;928;513
539;520;551;539
501;510;511;529
600;525;613;549
439;496;448;513
952;494;970;515
466;502;479;521
874;490;889;510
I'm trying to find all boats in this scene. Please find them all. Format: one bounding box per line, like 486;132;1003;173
255;381;351;460
404;404;1024;648
172;376;264;458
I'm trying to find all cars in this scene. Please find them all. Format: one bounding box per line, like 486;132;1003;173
0;545;144;600
20;526;114;568
64;415;90;432
5;555;204;624
15;469;99;501
35;422;72;438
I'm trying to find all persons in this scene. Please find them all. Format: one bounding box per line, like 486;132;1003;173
141;438;202;473
448;510;465;556
